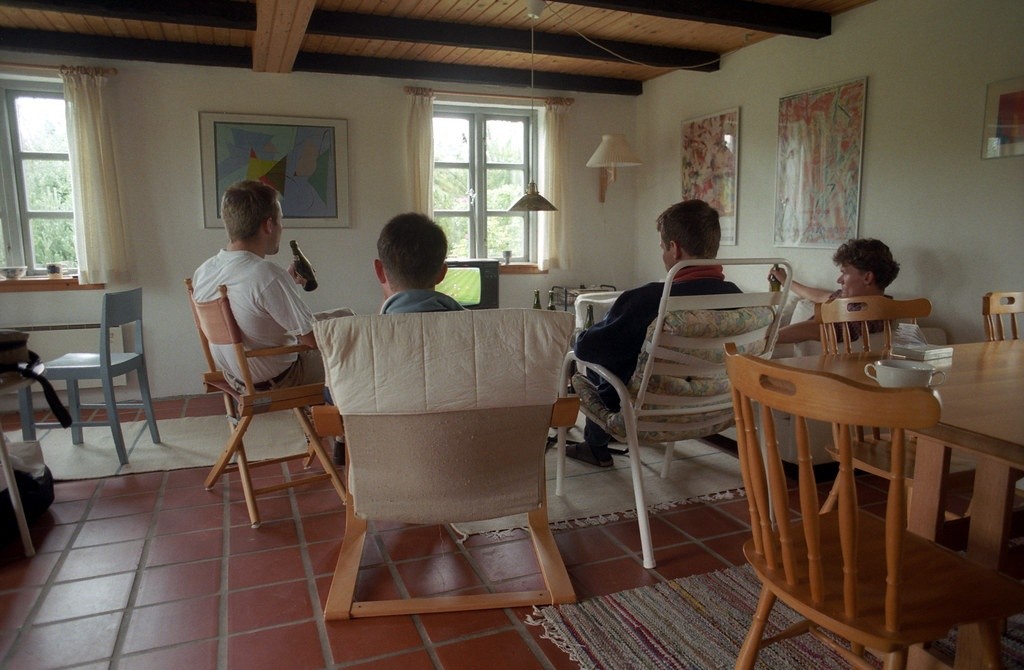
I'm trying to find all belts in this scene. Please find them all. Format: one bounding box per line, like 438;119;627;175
228;354;300;391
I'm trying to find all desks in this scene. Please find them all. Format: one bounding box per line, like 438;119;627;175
764;338;1024;670
0;349;45;558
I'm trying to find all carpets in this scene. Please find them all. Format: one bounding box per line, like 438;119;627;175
450;399;748;545
523;550;1024;670
3;408;308;481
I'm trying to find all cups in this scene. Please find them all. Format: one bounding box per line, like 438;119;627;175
864;359;946;387
47;264;63;279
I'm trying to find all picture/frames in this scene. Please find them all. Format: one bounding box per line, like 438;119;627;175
197;111;353;229
681;105;742;247
772;75;868;249
978;74;1024;161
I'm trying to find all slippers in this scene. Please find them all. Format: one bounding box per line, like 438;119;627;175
566;441;613;467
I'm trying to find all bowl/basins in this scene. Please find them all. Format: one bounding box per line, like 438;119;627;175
0;266;28;280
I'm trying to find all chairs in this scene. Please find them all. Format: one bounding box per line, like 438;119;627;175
818;293;976;526
961;292;1024;518
723;339;1024;670
185;278;348;530
554;258;793;571
19;286;161;466
310;307;578;620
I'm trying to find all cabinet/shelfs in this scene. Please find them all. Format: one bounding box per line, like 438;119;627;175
551;285;616;392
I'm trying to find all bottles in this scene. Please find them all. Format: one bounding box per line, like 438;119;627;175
547;291;556;310
532;289;542;309
769;264;781;292
583;304;595;331
289;240;318;292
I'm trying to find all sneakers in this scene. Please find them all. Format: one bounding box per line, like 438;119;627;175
333;441;345;465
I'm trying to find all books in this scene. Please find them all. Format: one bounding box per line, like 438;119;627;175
891;323;928;349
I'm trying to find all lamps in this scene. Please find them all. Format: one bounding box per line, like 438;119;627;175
508;0;560;212
586;133;643;205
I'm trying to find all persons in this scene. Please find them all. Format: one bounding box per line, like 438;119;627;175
374;212;467;314
766;238;900;344
192;179;358;466
564;198;744;467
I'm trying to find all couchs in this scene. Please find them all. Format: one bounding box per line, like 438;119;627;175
575;290;946;479
